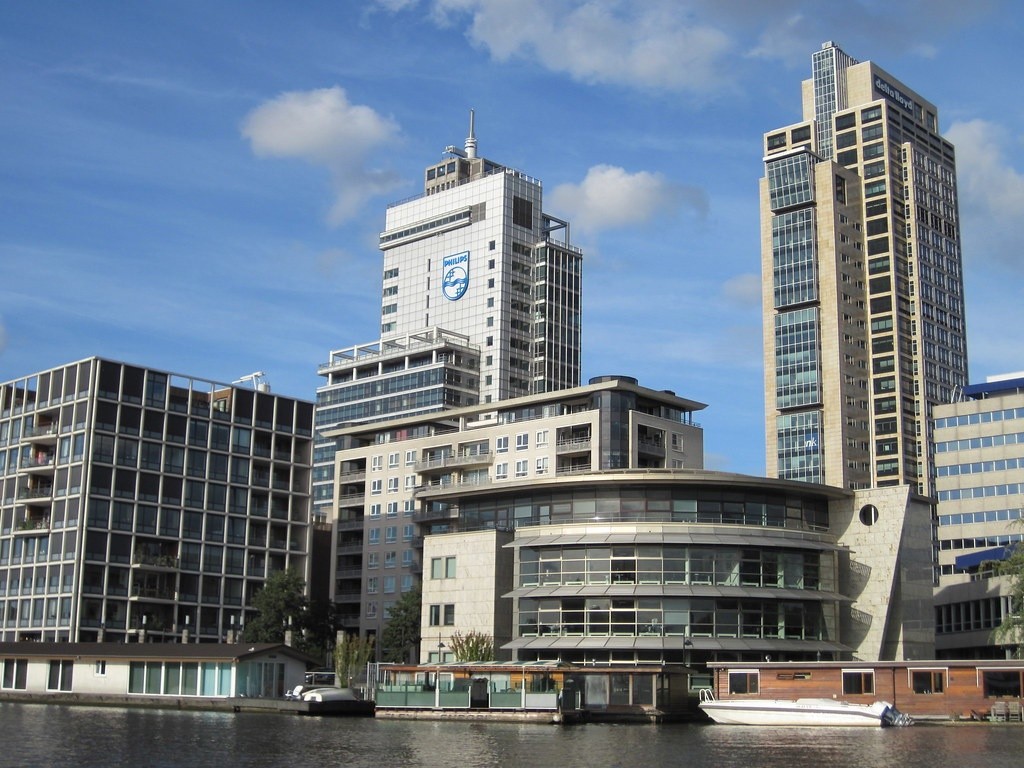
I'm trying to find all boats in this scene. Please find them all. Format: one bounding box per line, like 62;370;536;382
360;659;697;726
705;659;1024;723
696;689;912;729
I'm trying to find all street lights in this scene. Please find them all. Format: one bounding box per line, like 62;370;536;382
682;636;694;668
436;632;445;662
